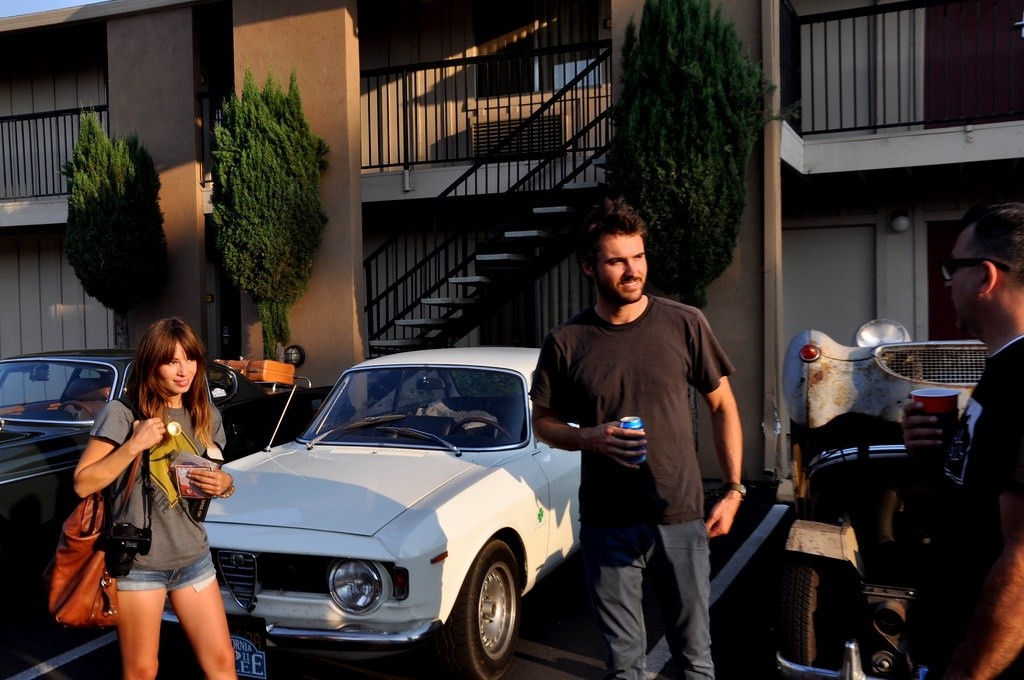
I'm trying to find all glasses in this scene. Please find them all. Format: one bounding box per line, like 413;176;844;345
941;254;1010;282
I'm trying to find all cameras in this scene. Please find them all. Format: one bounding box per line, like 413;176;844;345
98;521;152;579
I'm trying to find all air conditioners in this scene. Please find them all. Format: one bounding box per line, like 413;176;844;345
469;112;569;156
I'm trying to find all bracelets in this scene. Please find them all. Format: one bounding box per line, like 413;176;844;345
220;485;235;498
722;483;746;496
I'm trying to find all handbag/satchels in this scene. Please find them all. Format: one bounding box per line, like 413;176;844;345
47;490;122;625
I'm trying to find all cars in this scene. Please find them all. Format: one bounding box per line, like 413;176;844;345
774;320;994;679
159;344;598;680
0;348;370;680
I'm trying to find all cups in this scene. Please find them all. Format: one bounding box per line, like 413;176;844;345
912;388;960;455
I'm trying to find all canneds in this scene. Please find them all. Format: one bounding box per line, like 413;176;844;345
620;417;646;465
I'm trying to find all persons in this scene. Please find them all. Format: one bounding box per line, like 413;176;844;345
532;197;744;680
73;318;239;680
903;201;1024;680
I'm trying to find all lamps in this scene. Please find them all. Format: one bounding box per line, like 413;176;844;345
890;209;910;233
284;344;306;368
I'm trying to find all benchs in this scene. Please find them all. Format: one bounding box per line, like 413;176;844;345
420;394;525;442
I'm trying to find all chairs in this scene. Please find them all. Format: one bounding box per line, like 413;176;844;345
64;377;110;421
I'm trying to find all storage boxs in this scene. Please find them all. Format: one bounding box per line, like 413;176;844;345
210;359;296;385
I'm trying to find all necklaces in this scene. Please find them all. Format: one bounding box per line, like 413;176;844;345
167;414;181;436
